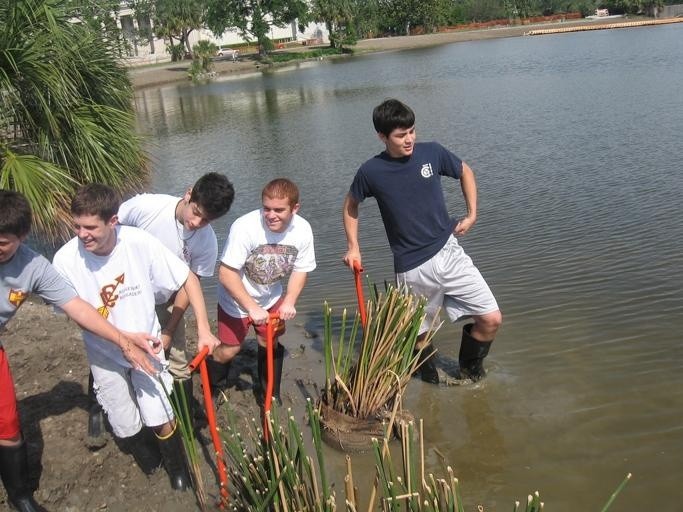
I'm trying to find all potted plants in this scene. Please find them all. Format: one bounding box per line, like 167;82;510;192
317;277;444;455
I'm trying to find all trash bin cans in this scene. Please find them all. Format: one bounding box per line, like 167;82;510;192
561;16;565;22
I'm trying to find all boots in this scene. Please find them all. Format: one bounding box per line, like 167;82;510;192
206;356;230;397
122;418;189;491
458;322;493;380
0;442;42;512
257;342;285;407
174;378;196;440
413;339;440;384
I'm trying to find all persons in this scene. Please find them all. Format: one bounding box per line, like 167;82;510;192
234;51;240;62
343;99;501;385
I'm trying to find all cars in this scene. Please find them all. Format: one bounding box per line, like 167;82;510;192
215;48;240;57
